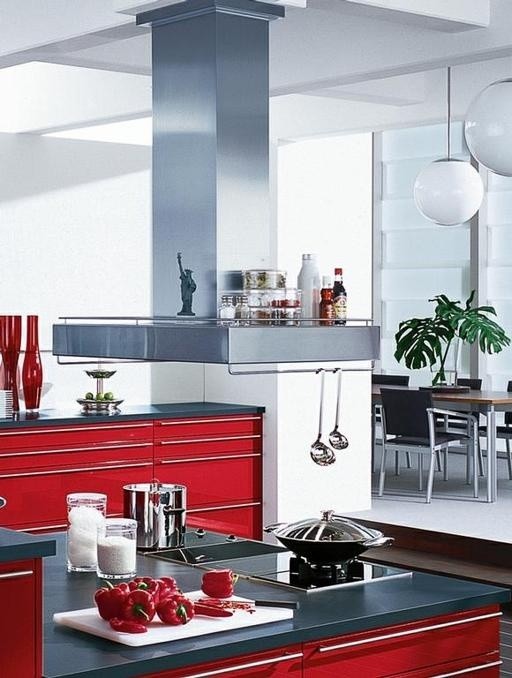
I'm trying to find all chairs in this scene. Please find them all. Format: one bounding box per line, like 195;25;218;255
372;369;512;503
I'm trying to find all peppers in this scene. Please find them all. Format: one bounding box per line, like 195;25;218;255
93;568;235;633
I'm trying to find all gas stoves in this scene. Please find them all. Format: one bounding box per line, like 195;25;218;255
143;528;413;594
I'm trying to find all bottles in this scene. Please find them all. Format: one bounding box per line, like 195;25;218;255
66;493;108;572
1;315;44;419
220;252;347;326
97;517;138;580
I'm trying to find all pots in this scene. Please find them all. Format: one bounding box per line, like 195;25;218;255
123;476;188;551
264;510;395;565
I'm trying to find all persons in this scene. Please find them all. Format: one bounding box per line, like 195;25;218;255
175;250;197;315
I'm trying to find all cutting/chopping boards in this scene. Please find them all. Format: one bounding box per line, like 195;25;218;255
52;588;293;649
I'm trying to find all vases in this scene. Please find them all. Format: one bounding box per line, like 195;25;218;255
22;315;43;414
0;315;21;413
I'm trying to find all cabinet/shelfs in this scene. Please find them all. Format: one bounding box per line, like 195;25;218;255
1;401;265;546
303;604;504;678
145;644;304;678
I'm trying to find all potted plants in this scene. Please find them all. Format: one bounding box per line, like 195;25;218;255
394;290;509;391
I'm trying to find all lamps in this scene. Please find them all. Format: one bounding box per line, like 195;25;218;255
414;63;484;228
465;77;512;178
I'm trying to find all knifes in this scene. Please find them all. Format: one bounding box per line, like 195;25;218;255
194;597;298;610
